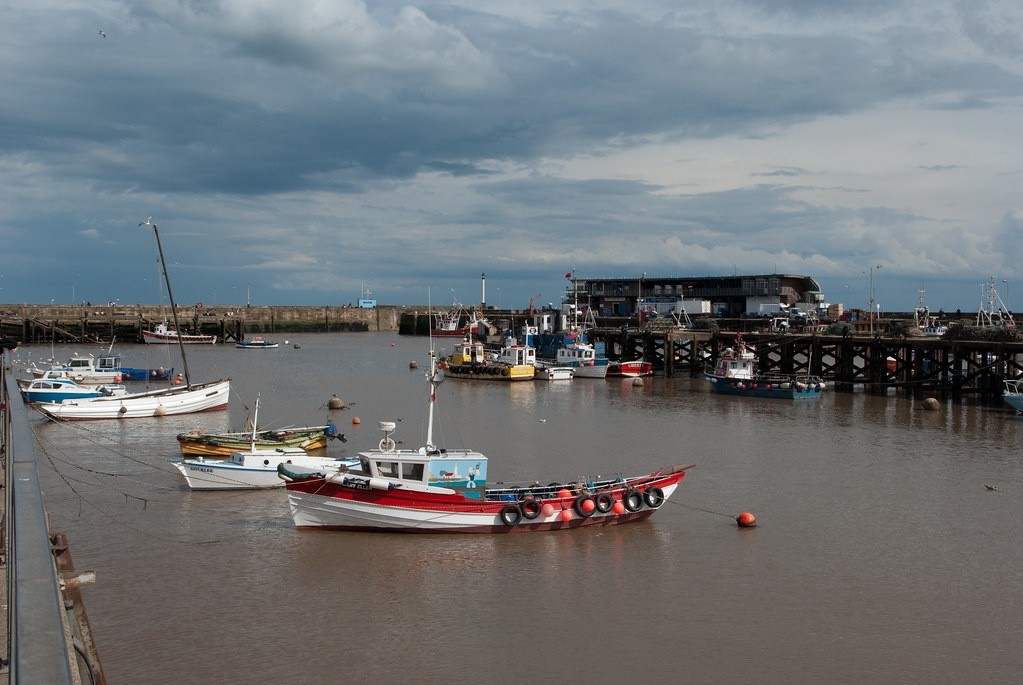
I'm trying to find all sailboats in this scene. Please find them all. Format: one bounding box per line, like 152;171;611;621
30;223;235;422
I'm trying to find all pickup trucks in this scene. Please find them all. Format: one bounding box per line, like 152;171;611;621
771;307;807;320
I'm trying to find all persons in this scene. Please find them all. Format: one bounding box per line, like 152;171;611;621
579;362;584;367
589;359;594;366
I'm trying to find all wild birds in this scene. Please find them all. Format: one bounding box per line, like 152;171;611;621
348;402;356;405
137;216;152;227
984;484;999;491
397;418;404;422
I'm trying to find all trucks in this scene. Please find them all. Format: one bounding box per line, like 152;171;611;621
713;303;729;317
560;303;583;316
670;300;711;318
759;303;790;319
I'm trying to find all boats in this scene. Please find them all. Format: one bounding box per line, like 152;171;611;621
976;353;1008;370
430;301;485;338
1001;380;1023;413
702;333;826;400
885;355;897;372
275;286;696;534
141;315;217;344
236;337;279;349
176;412;348;457
17;344;174;404
433;273;655;381
171;392;382;492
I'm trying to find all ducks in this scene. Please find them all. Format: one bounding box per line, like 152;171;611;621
536;417;546;422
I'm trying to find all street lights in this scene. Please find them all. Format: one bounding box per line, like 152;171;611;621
870;265;884;338
1003;279;1009;298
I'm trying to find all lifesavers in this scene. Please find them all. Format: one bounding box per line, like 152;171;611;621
155;335;213;341
501;504;522;526
644;486;664;507
573;494;596;517
623;490;644;511
530;328;536;334
491;481;578;499
594;493;613;513
449;366;508;376
522;499;541;519
